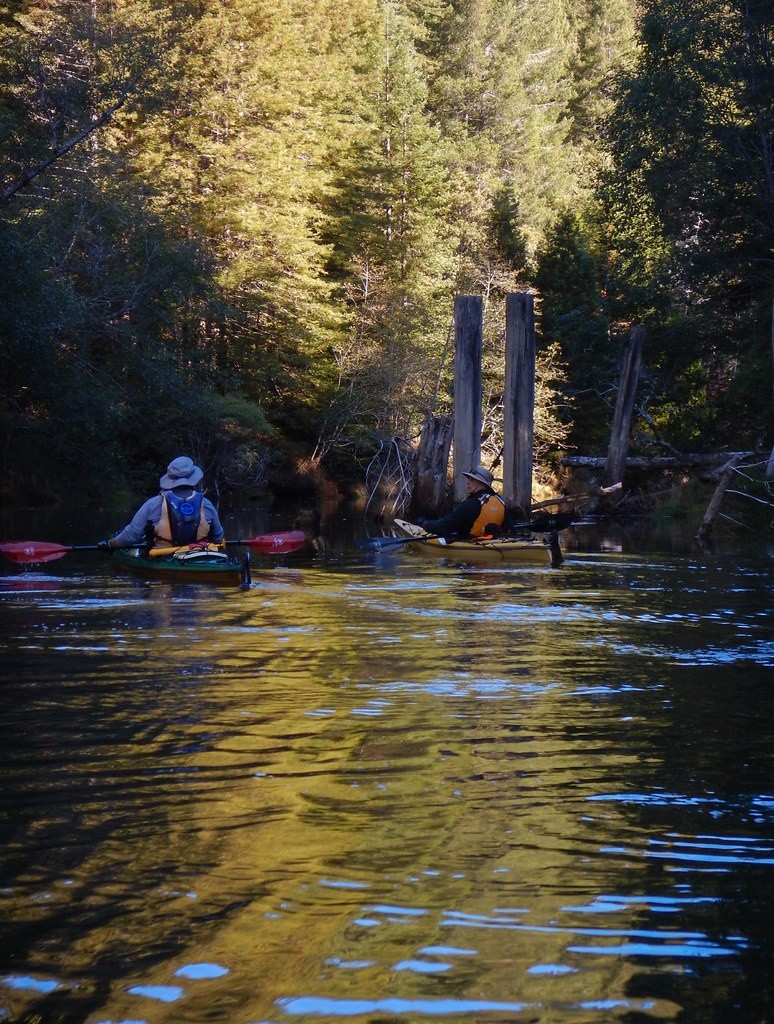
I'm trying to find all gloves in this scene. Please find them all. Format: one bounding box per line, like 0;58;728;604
97;538;111;549
417;517;426;528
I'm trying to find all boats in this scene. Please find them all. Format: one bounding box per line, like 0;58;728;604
40;533;256;591
391;516;566;567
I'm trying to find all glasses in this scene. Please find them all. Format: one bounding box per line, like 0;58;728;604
469;468;489;482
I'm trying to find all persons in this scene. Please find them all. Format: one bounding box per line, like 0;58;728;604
414;467;510;543
97;457;223;553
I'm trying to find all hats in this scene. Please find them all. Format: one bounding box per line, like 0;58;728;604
159;456;203;490
462;466;494;487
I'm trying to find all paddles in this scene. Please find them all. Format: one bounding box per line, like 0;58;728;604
357;524;545;556
1;528;313;564
